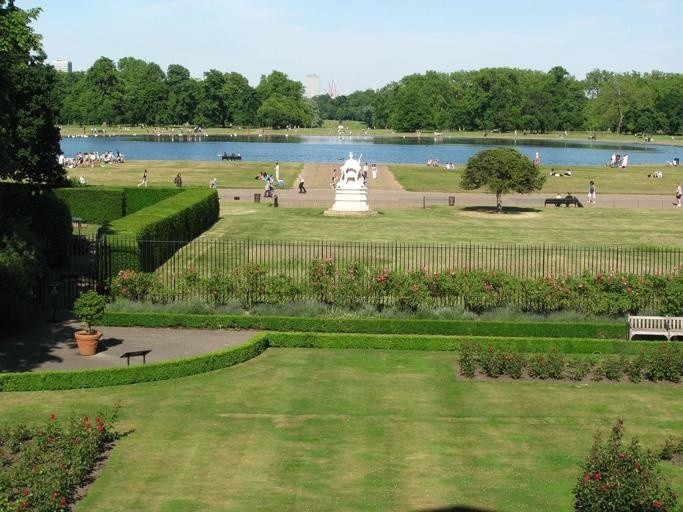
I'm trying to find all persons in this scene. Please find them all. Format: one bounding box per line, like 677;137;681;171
665;159;678;167
427;158;454;169
64;175;88;184
587;181;596;203
634;131;654;143
224;151;241;162
57;150;125;168
175;173;182;187
650;171;663;177
256;161;307;204
286;123;598;139
675;183;683;204
138;169;148;188
361;162;377;184
330;170;339;191
550;167;572;177
606;152;629;168
211;178;217;188
91;123;202;136
558;192;572;205
532;158;541;165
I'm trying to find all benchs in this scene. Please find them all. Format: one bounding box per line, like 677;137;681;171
545;196;577;207
626;312;683;341
222;155;241;162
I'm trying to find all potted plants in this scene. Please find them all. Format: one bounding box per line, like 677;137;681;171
72;289;107;356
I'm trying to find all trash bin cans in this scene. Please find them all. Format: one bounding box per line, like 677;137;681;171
255;194;261;202
448;196;455;206
